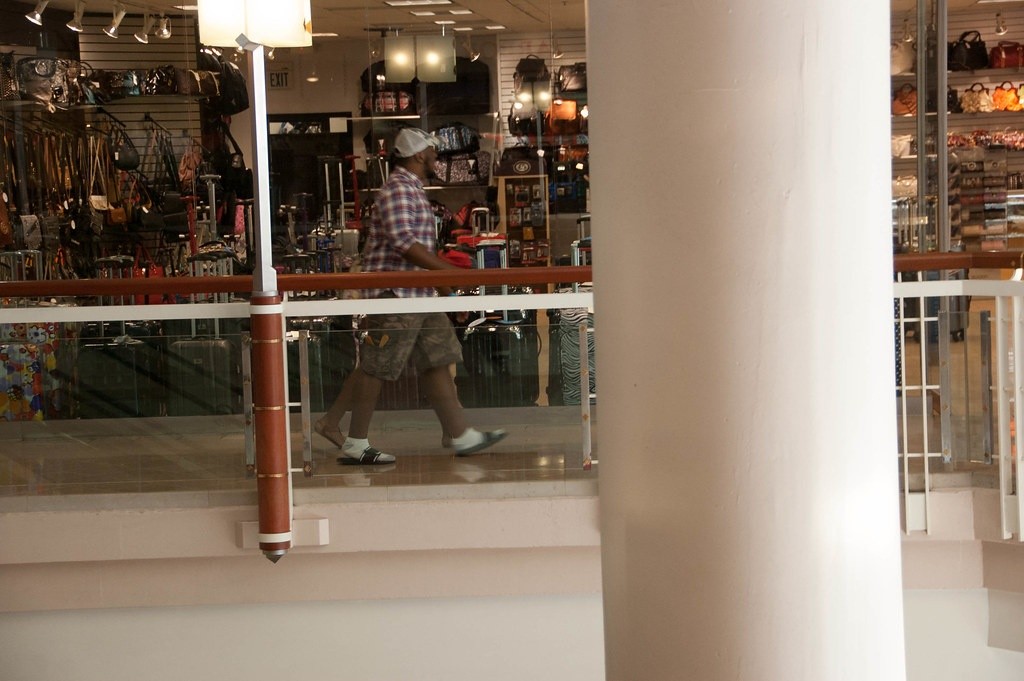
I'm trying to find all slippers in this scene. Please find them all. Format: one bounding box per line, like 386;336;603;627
335;446;396;465
453;428;506;456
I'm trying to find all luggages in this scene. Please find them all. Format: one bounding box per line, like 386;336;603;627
0;230;597;422
893;241;972;343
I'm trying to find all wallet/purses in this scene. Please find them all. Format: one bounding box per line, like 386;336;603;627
948;147;1024;252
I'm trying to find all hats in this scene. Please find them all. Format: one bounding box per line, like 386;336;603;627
392;127;440;157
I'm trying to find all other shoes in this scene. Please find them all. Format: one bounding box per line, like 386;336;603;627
441;434;454;448
314;419;345;449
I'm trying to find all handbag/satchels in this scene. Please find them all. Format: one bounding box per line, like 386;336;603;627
0;45;255;253
892;134;914;157
947;31;1024;71
947;81;1024;113
893;176;918;198
891;19;916;76
346;54;592;243
947;126;1024;154
891;83;917;114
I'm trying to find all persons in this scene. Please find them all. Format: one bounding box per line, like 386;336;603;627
314;128;511;464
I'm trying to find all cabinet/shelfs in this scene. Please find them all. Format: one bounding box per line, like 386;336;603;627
890;69;953;160
346;112;499;191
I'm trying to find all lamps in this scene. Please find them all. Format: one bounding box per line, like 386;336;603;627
384;28;416;83
415;23;457;83
552;37;564;59
155;12;171;40
25;0;51;26
134;10;156;44
66;0;87;32
103;0;128;39
462;41;481;62
